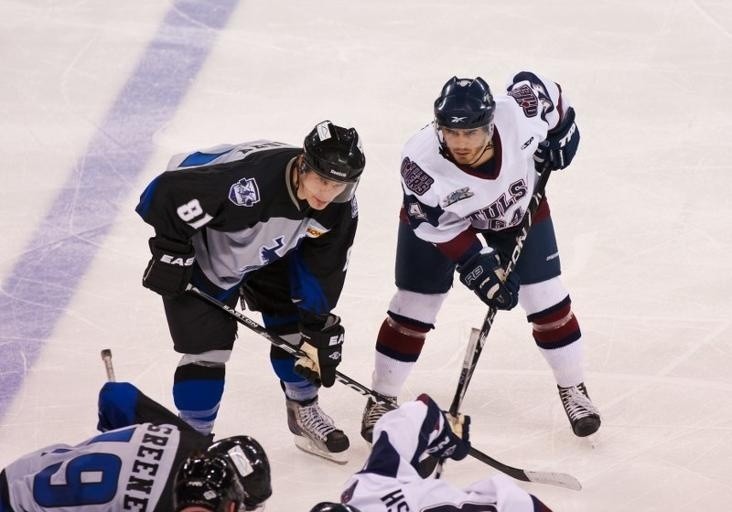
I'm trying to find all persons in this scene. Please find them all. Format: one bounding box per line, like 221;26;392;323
309;394;554;512
173;448;245;511
1;382;274;511
359;70;601;439
134;119;365;466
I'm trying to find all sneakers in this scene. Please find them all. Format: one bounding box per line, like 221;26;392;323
557;383;600;439
360;390;397;445
285;395;352;455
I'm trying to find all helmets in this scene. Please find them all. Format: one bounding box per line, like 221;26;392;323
176;436;272;511
301;120;366;204
434;76;496;150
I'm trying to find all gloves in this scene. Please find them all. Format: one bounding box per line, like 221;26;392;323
534;107;581;172
459;246;520;310
293;314;344;388
417;392;470;461
142;236;201;300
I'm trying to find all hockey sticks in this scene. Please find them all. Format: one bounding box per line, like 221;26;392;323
185;283;583;492
